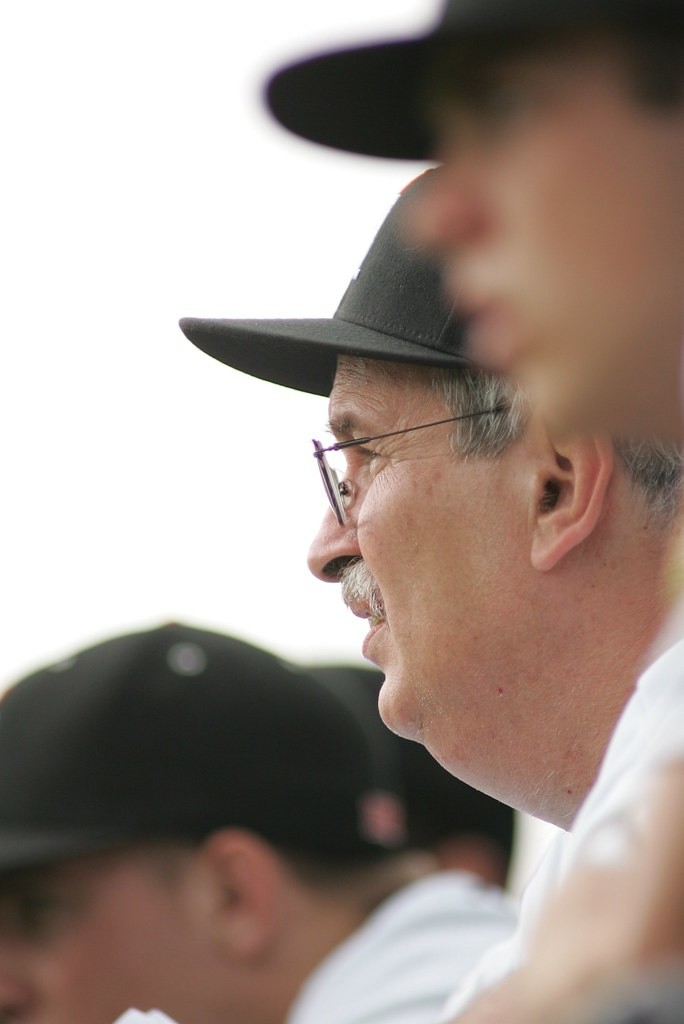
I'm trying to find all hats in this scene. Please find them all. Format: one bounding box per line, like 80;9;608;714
300;664;513;857
180;170;506;398
0;624;414;873
266;0;684;165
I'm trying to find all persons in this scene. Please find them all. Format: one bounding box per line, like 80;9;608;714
0;621;516;1024
262;0;684;1024
186;174;684;831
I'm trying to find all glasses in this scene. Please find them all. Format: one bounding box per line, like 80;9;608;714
310;411;497;527
